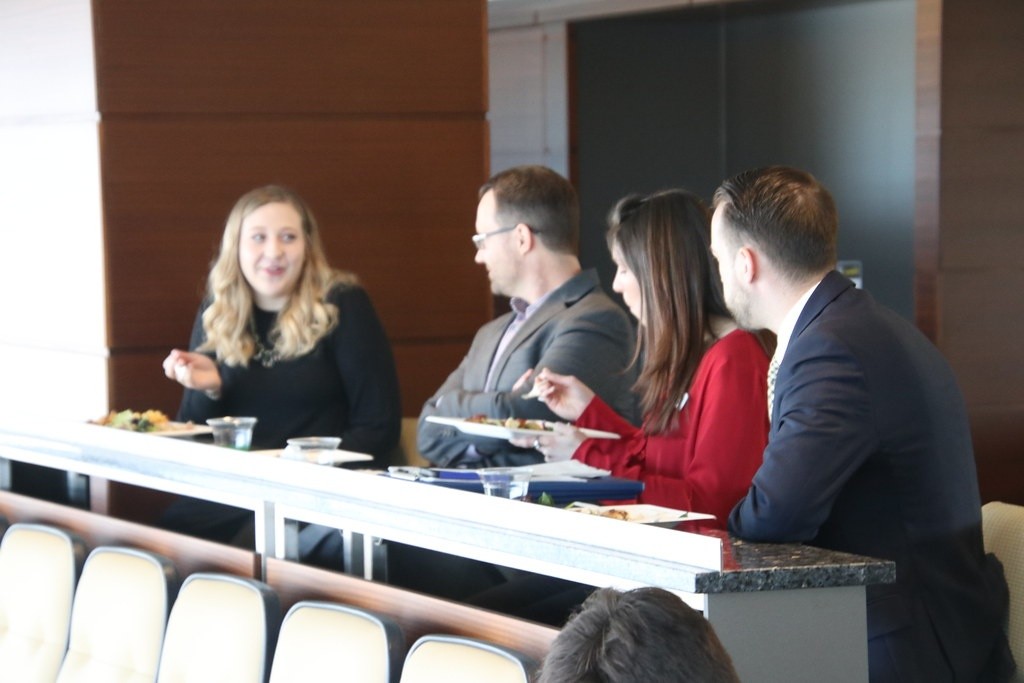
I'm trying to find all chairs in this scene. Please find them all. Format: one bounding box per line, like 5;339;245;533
0;518;87;683
983;499;1024;683
399;633;529;683
268;600;390;683
56;543;178;683
157;572;280;683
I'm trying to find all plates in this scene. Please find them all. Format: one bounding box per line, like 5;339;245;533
426;415;621;440
565;504;716;528
255;449;373;463
82;420;211;437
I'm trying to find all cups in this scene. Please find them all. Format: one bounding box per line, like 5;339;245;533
285;437;342;466
206;417;257;450
474;467;534;501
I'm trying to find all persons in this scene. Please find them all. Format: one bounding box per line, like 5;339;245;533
507;183;772;631
161;184;403;547
535;587;742;683
303;167;646;617
710;162;1023;683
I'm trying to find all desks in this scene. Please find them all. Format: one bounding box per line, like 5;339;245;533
0;411;895;683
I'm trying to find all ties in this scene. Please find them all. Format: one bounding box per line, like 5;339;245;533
766;355;780;424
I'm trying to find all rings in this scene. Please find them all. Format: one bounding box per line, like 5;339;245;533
533;437;541;448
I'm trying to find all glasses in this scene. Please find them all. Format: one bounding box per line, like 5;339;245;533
472;225;542;249
